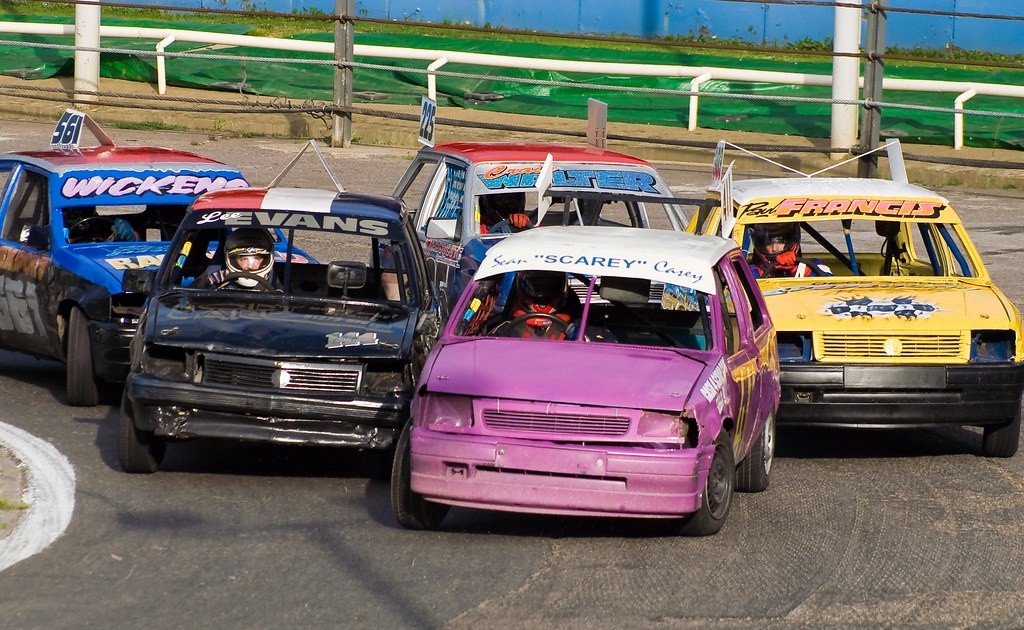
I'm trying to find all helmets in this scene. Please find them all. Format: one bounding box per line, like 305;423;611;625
515;269;569;315
753;222;801;269
224;227;274;287
487;192;526;220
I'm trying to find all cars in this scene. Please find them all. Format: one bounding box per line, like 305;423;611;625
120;186;445;475
390;155;783;537
687;177;1023;460
0;146;325;408
371;142;688;327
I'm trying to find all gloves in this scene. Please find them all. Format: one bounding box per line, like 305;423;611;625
111;218;136;241
774;251;798;271
207;269;231;289
508;213;530;228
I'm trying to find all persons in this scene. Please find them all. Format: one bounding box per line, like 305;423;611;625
748;222;834;278
479;192;538;234
189;228;286;293
479;270;590;342
65;207;142;241
